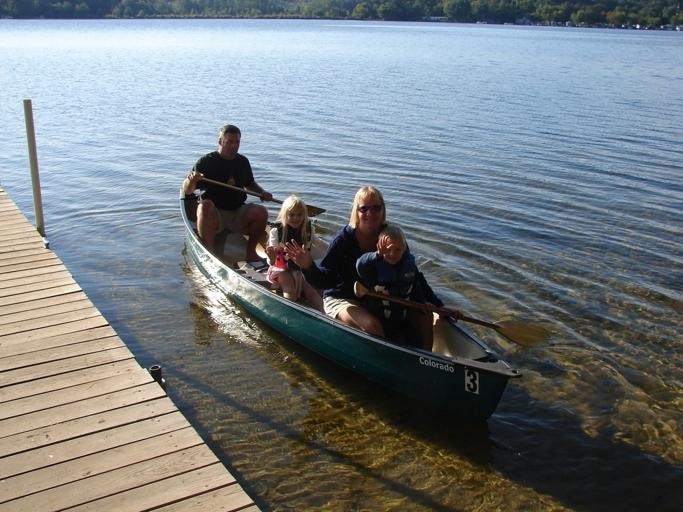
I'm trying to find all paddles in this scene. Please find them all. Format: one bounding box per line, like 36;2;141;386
200;177;326;217
364;291;550;348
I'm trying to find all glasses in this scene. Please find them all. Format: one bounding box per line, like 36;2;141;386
356;204;384;214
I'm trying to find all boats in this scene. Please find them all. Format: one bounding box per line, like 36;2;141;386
179;174;524;423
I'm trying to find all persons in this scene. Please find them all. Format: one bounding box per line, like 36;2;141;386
282;183;465;354
336;224;437;351
265;193;325;314
180;124;273;267
